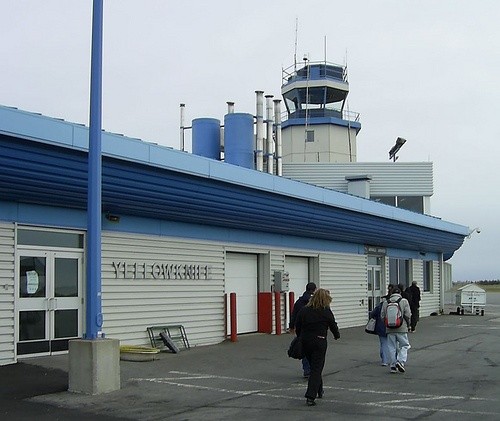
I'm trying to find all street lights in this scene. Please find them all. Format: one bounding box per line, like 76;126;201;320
388;136;407;162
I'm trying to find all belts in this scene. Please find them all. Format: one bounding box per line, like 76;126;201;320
317;335;325;339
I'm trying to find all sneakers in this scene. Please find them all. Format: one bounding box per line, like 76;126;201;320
390;367;398;374
395;362;405;373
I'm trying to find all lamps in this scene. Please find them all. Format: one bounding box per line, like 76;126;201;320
389;137;406;162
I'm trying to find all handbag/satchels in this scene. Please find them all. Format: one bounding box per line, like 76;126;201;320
288;336;306;360
365;318;378;335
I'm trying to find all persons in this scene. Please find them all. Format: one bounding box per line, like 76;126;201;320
367;282;421;375
293;288;340;406
291;281;321;378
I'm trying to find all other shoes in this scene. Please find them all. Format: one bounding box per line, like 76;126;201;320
411;329;416;333
318;391;324;399
303;373;310;377
306;398;317;406
380;363;390;366
407;326;411;332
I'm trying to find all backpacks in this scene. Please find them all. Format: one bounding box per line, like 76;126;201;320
384;298;403;329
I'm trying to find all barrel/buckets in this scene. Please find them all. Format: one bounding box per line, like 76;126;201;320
224;112;256;170
191;118;221;160
